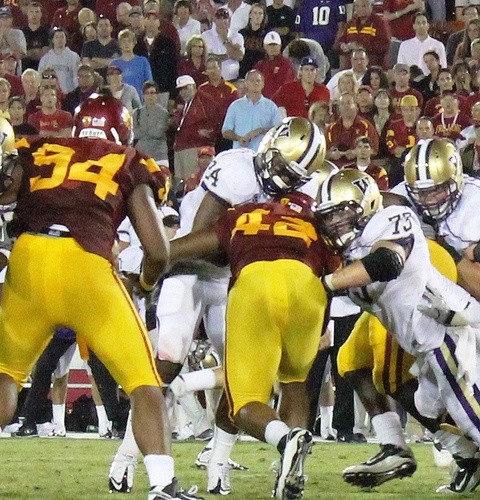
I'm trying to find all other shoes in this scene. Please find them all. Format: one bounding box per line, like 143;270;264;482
46;430;66;437
337;430;358;442
110;428;124;441
15;424;38;437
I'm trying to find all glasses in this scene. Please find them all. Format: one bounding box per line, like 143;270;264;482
41;75;57;79
216;9;229;16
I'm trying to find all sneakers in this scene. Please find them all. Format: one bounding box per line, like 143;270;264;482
344;442;416;489
273;427;312;500
147;477;202;500
437;452;480;495
206;457;232;496
196;449;246;471
108;454;135;495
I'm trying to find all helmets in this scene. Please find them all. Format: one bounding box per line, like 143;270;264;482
401;139;464;223
312;171;382;250
72;94;133;147
257;116;326;195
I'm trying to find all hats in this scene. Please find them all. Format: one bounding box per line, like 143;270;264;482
197;147;213;157
394;64;409;73
357;86;371;94
301;57;319;69
128;7;142;16
263;31;281;45
0;6;12;17
176;75;194;89
400;95;417;108
145;11;158;17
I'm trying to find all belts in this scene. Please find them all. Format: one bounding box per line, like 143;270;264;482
30;226;72;237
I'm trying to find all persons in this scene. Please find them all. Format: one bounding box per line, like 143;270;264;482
269;55;330;126
164;74;220;189
304;168;480;496
0;0;480;500
131;79;170;169
130;160;347;500
221;69;282;153
0;92;204;500
